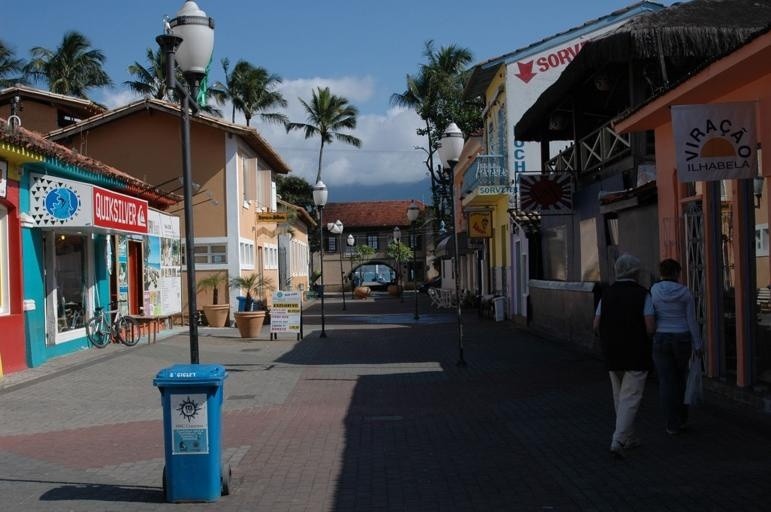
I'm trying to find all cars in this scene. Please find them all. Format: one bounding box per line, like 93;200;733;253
417;275;442;295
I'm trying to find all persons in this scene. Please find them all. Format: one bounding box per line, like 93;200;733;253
592;254;657;461
649;257;705;437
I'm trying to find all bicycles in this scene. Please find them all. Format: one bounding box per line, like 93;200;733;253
87;299;141;347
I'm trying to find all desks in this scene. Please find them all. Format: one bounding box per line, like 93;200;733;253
125;311;186;345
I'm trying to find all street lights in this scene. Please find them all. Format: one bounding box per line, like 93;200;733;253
346;232;354;300
326;219;349;311
314;178;328;338
158;0;234;366
438;120;466;366
392;226;404;303
406;198;420;320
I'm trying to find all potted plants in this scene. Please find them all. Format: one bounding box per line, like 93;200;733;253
193;264;232;329
346;239;373;300
382;237;415;298
225;271;277;341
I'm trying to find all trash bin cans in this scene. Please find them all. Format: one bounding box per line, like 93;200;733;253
234;296;254;328
152;363;232;503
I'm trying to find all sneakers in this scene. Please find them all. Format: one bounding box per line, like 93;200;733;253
611;441;628;461
627;439;641;449
